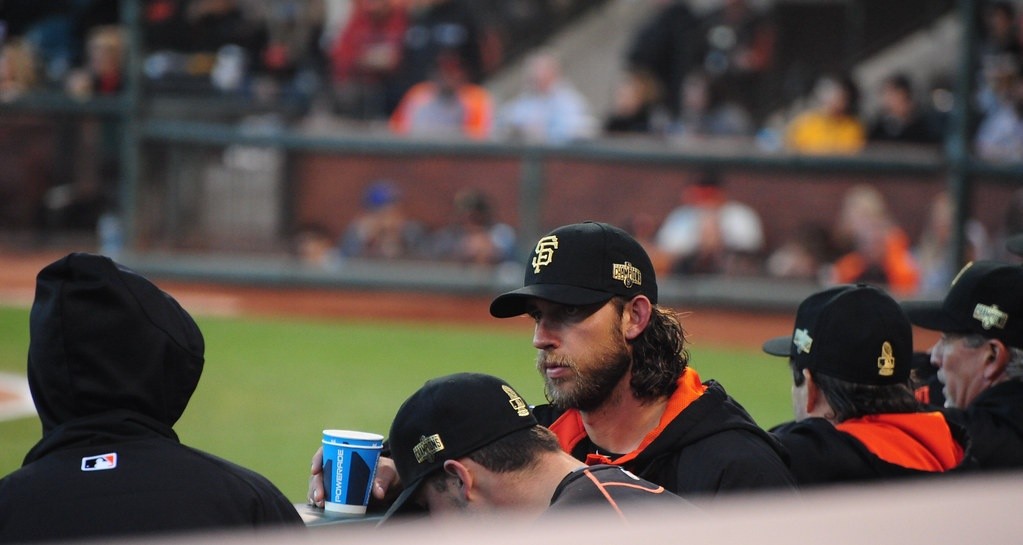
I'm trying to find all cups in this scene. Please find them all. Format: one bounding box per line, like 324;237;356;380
322;430;383;516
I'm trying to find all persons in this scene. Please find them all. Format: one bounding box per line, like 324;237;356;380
759;260;1023;488
1;252;306;545
305;220;801;511
0;0;1022;298
381;371;708;520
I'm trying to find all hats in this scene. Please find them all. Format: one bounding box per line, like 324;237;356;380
762;284;913;384
373;373;537;527
491;221;659;318
1006;234;1023;257
900;261;1023;348
